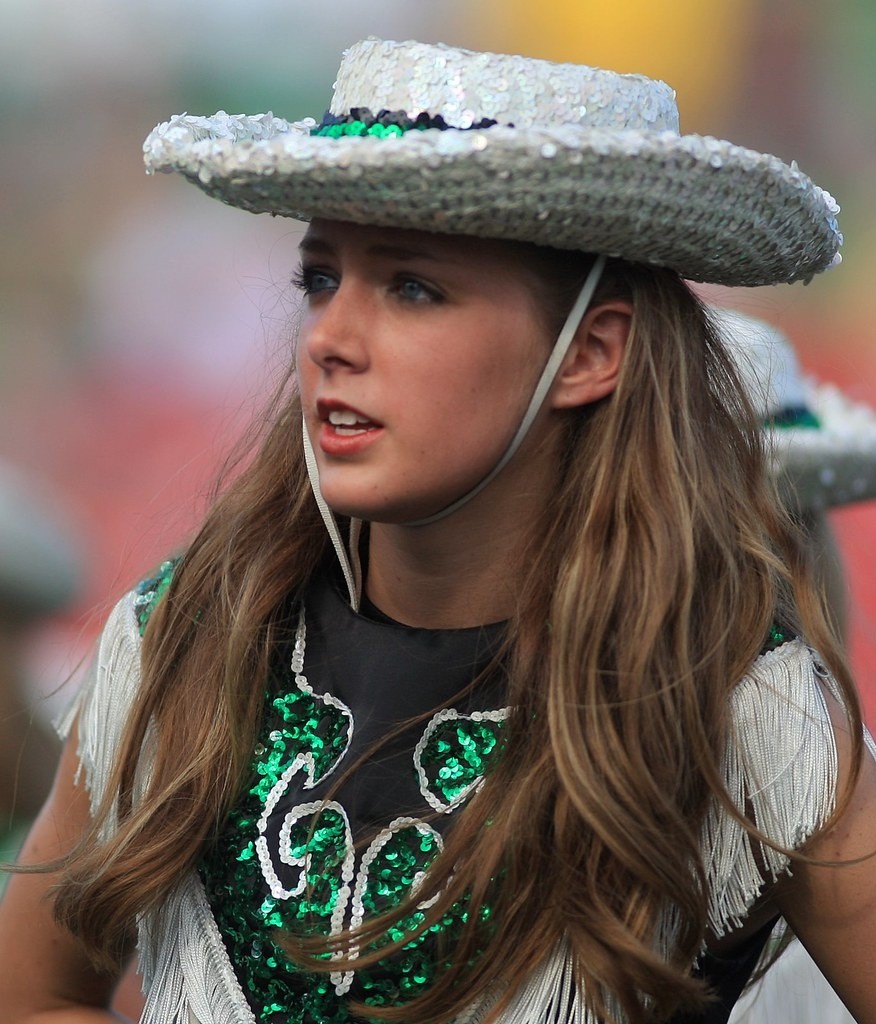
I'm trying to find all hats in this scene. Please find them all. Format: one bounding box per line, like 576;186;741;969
140;37;841;288
703;305;876;509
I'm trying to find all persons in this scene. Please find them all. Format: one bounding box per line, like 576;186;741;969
0;34;876;1024
